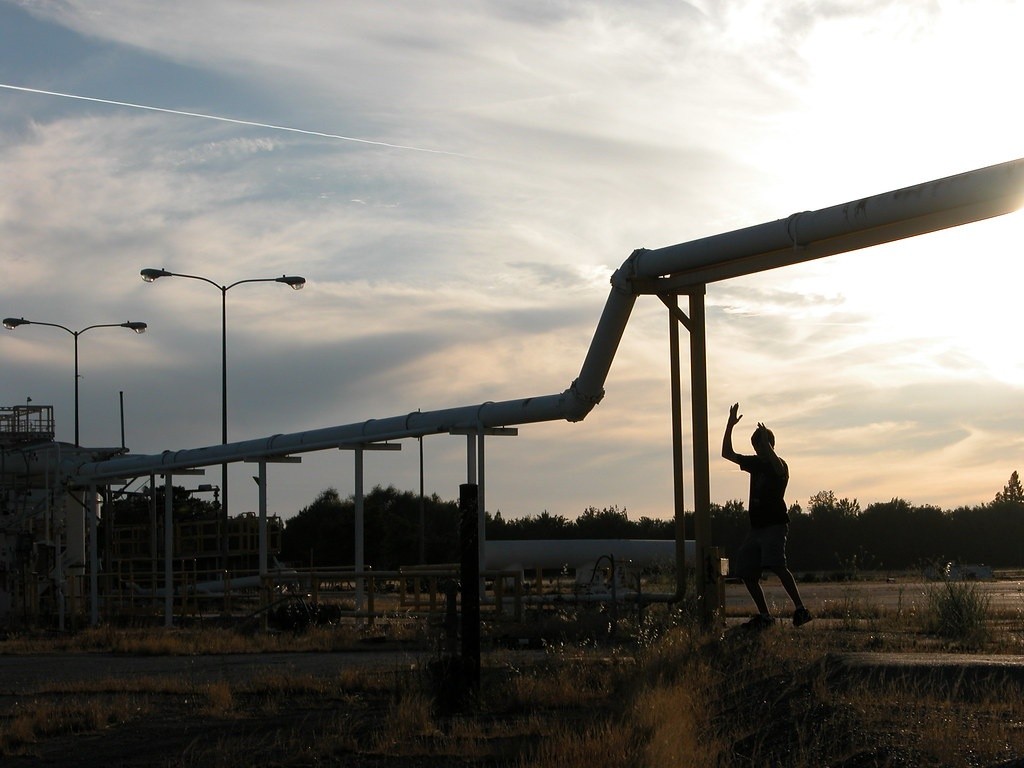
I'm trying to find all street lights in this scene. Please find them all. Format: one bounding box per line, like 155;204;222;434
2;317;148;457
141;268;305;590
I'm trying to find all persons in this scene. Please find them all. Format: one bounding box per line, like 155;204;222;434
721;402;812;630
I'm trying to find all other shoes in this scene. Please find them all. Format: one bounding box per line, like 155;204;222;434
741;614;776;630
792;608;812;627
